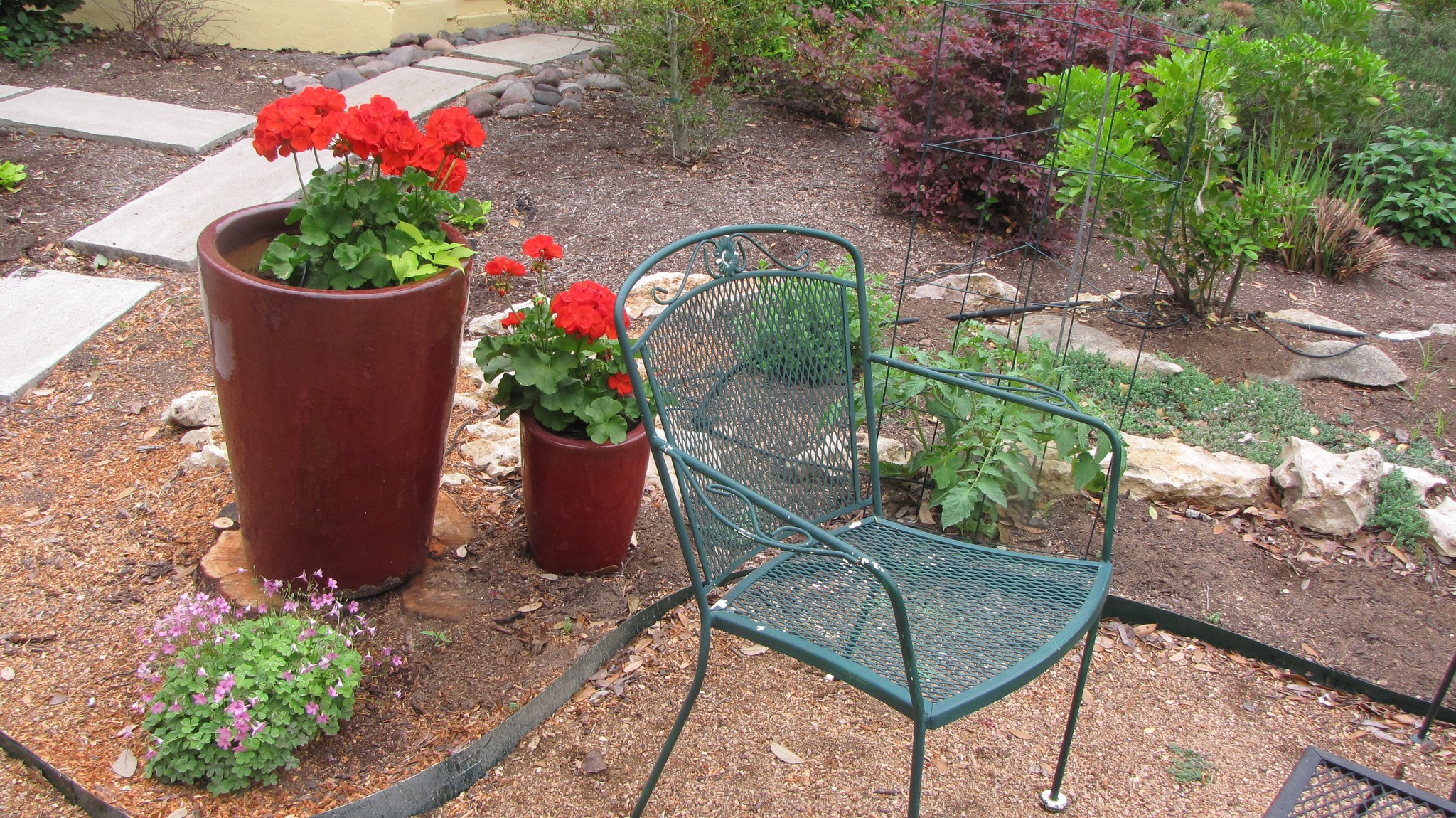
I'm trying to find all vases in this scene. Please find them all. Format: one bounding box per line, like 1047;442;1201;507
196;197;474;603
513;395;660;581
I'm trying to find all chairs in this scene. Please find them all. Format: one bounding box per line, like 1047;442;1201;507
614;221;1125;818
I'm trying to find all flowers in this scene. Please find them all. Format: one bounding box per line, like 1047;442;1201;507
474;230;682;443
250;87;491;291
